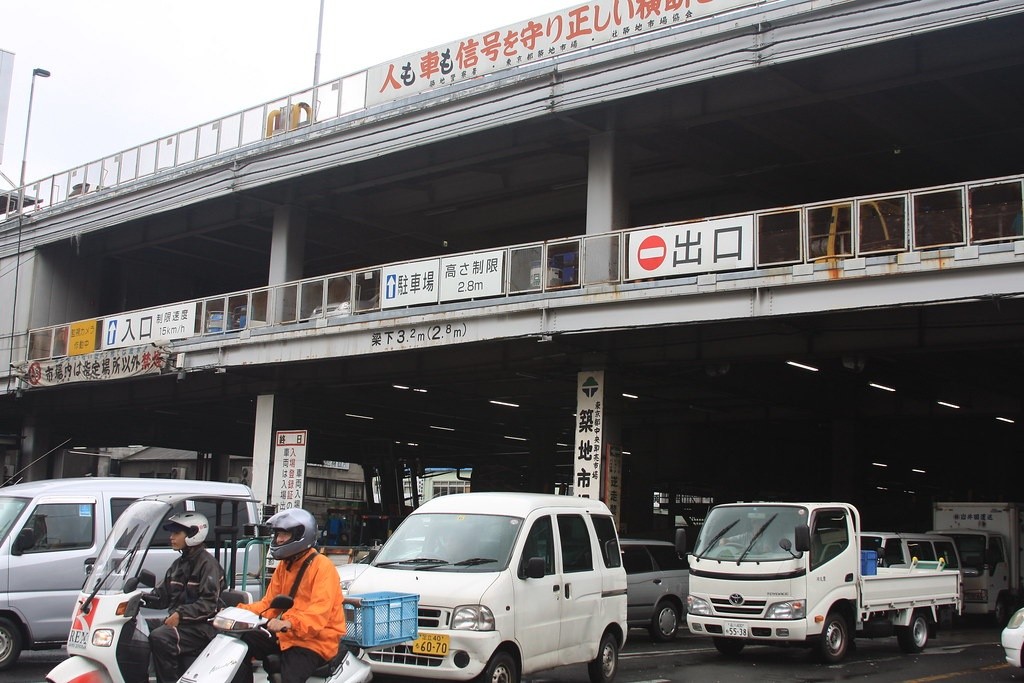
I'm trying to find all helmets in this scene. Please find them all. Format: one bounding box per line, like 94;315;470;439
168;511;209;546
265;508;317;560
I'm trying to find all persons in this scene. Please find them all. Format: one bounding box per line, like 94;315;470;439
718;519;773;552
139;512;224;683
232;508;346;683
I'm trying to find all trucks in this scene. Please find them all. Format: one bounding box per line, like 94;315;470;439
686;502;961;663
933;502;1024;628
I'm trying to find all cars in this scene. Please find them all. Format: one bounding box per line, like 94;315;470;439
618;538;689;642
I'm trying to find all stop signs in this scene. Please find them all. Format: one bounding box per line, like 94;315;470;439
637;235;667;271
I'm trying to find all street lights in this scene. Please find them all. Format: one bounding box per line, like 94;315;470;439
15;67;51;216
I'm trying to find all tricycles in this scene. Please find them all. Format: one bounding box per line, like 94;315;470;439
46;492;266;683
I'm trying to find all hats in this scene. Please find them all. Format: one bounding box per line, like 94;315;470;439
163;523;189;531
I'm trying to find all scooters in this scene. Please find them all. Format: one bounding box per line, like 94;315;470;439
176;575;374;683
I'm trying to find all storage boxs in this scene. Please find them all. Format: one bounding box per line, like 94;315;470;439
860;550;878;576
344;589;420;645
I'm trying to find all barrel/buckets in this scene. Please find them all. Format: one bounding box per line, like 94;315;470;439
529;260;541;289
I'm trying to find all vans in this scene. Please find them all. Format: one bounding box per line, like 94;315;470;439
855;531;961;616
0;477;262;672
335;492;629;683
